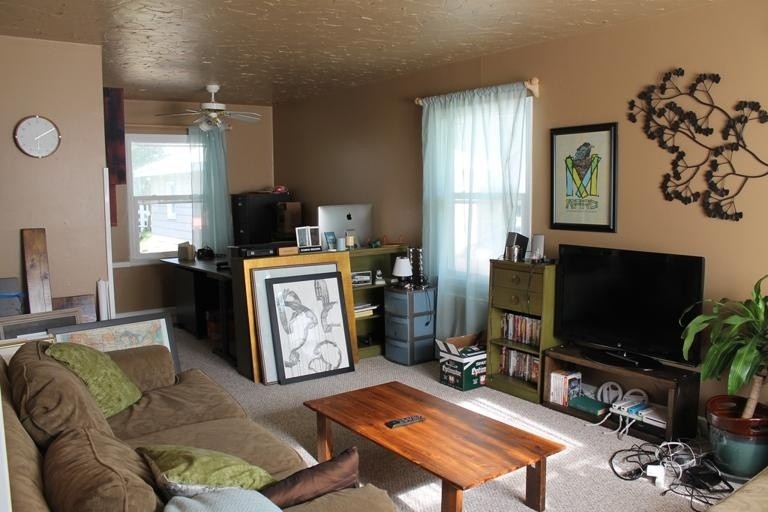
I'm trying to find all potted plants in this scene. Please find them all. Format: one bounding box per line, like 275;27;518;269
672;272;766;481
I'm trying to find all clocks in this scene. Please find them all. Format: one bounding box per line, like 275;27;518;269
14;115;62;159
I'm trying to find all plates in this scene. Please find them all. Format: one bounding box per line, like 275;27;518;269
327;248;337;252
335;248;348;251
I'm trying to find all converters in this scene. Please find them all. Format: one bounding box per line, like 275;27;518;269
645;464;663;477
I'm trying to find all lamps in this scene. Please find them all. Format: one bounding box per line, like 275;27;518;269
191;112;233;133
390;254;414;286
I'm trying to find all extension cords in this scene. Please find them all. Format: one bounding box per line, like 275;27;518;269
655;466;665;487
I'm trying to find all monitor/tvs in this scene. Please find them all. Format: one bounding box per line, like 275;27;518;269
316;203;373;250
552;243;706;373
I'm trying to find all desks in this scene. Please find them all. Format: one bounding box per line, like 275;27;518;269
160;253;233;364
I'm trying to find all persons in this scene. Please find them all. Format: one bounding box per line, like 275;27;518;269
571;142;592;179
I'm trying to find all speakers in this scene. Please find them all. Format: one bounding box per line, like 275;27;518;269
532;234;544;263
504;232;529;258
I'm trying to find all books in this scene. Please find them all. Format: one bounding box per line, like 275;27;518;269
498;312;541;389
354;304;379;318
549;370;609;416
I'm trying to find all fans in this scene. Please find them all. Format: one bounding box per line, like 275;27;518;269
151;83;263;133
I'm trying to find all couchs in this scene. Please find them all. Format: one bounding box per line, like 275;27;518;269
1;342;408;511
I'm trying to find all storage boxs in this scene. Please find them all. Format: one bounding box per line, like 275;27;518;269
436;334;485;391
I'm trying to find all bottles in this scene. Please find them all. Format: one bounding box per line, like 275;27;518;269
525;248;556;265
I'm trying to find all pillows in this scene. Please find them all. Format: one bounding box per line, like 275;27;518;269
43;429;162;511
134;444;278;501
166;486;286;512
105;345;174;392
44;342;145;418
259;442;358;507
4;349;115;452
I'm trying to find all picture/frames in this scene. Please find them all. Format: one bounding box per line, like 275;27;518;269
548;121;617;233
262;271;354;385
0;307;84;338
45;312;182;375
0;334;55;357
248;262;336;384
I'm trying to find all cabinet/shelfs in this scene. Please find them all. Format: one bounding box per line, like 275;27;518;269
230;192;302;247
483;255;557;404
385;284;438;364
228;242;409;382
541;340;698;447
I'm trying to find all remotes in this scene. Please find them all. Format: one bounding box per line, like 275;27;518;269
385;415;425;429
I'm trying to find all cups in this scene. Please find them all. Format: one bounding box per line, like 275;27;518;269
177;241;196;264
504;247;519;263
336;238;345;249
346;235;354;247
265;184;274;192
275;185;289;193
